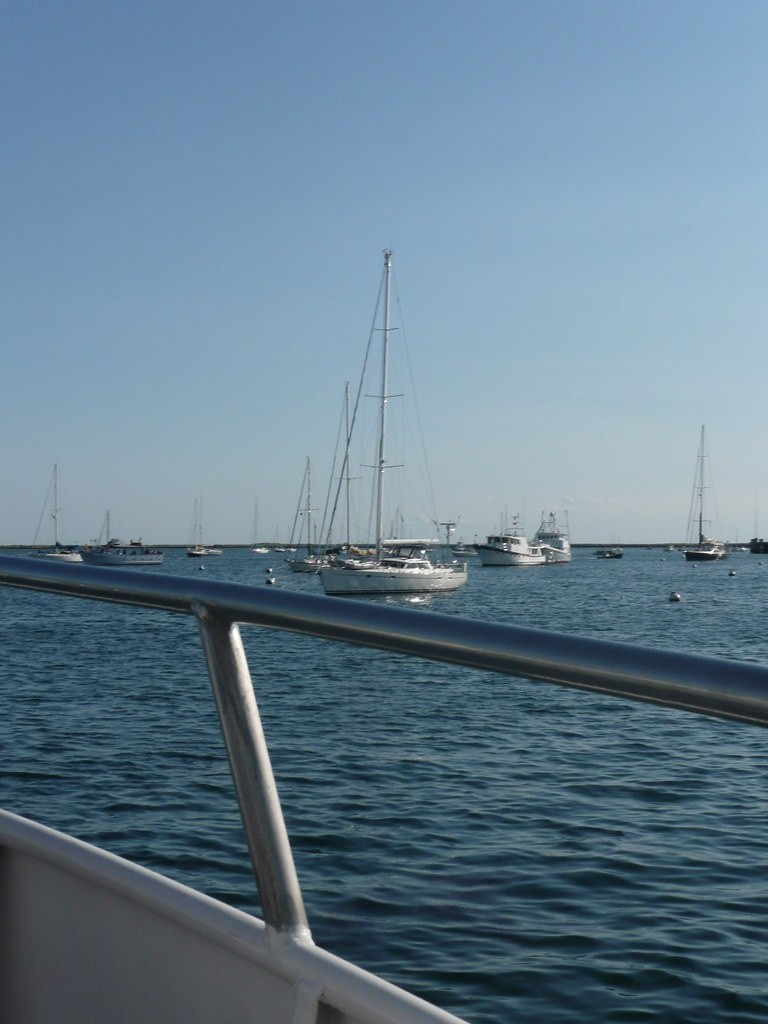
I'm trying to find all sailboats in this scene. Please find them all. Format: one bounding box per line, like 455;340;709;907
186;494;223;556
283;457;331;573
84;510;165;564
251;497;297;553
27;462;84;562
317;248;470;595
683;423;732;561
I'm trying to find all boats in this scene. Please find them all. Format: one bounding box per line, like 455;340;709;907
475;511;546;566
530;513;571;563
593;549;623;558
663;545;688;552
451;537;478;557
